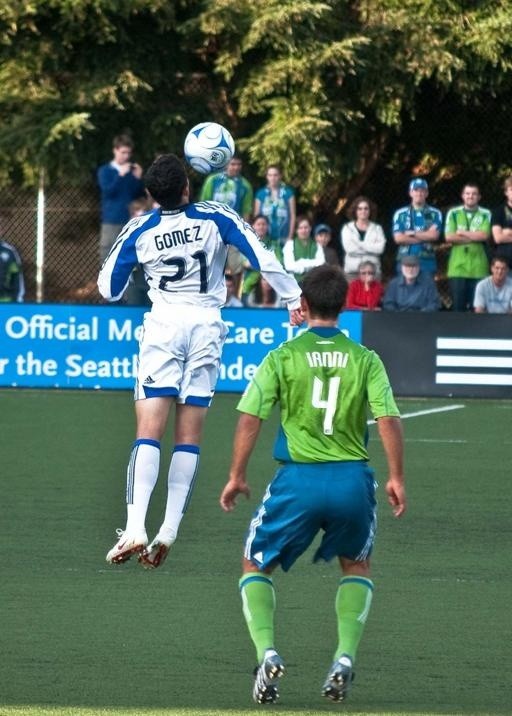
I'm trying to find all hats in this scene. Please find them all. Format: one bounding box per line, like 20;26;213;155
409;178;428;190
314;223;332;234
400;256;419;266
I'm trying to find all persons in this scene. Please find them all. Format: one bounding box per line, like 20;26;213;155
218;259;409;707
92;133;143;266
95;154;305;573
0;242;27;304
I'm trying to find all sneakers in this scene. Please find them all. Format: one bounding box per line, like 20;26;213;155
137;534;172;571
252;646;286;705
321;652;355;703
104;529;149;565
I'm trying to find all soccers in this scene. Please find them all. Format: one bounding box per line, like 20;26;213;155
182;120;234;171
